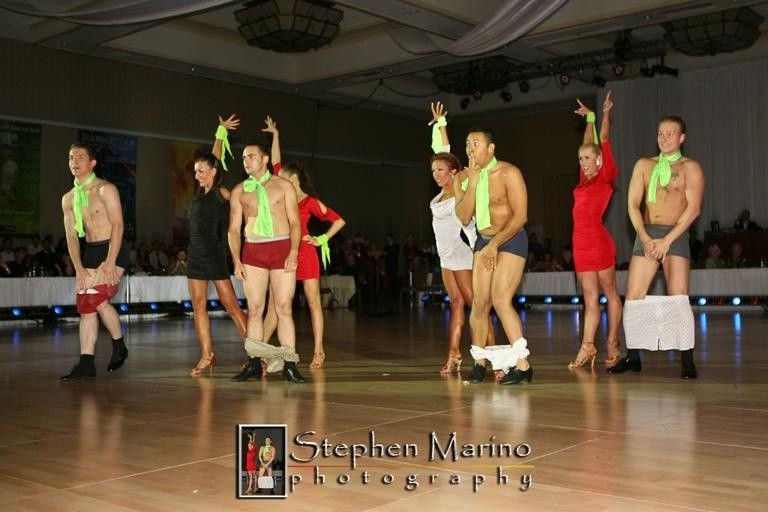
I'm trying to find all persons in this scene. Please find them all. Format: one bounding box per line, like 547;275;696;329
187;112;268;378
607;115;704;380
427;101;506;379
0;234;76;278
690;208;768;270
226;142;307;383
255;436;275;495
567;88;623;369
524;232;575;271
245;433;257;493
454;122;533;384
309;224;444;314
262;116;345;369
61;141;128;381
124;238;189;276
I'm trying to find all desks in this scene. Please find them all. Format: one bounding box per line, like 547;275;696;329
127;275;247;322
517;267;768;310
0;275;128;327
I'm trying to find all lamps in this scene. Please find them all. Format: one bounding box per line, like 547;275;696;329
460;52;679;111
661;6;765;57
231;0;344;53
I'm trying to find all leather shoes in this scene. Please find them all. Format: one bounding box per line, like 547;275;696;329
270;489;275;495
106;347;129;373
58;365;97;386
681;357;698;380
498;365;534;385
256;488;262;494
462;363;488;384
282;359;308;384
606;356;641;376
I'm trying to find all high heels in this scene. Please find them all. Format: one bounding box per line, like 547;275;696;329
440;355;463;374
310;352;326;370
245;488;252;493
191;351;217;374
253;488;258;493
604;339;621;368
568;342;597;368
232;357;263;381
493;369;508;381
